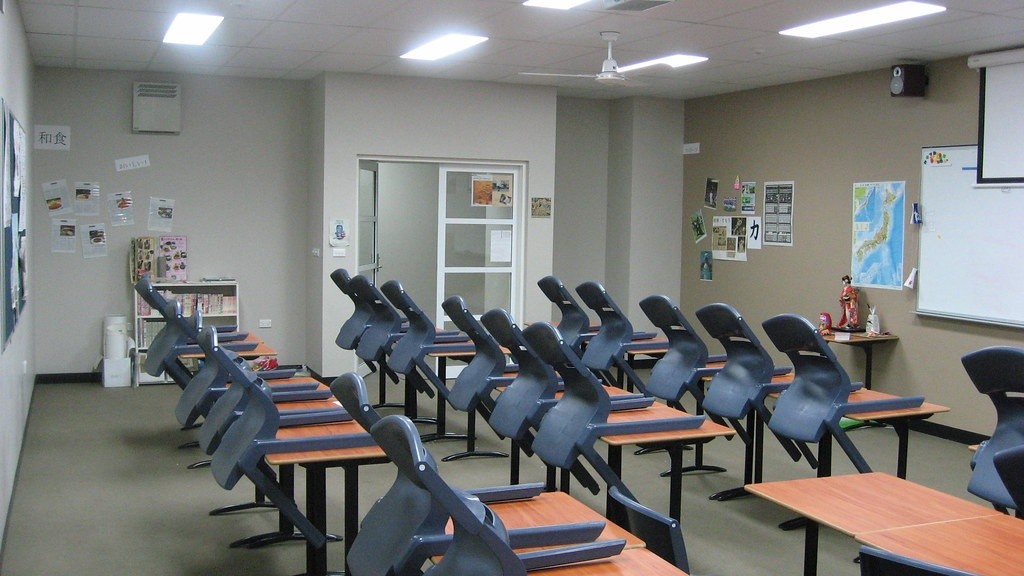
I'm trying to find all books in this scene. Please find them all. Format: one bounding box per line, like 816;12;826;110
130;236;157;283
138;290;237;382
200;277;239;284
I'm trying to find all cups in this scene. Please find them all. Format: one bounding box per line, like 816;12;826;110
866;314;880;335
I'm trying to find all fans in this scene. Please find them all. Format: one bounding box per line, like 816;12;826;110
515;31;672;87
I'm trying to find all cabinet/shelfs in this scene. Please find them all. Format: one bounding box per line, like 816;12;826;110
133;281;239;384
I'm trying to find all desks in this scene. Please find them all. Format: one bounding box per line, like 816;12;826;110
177;313;1024;576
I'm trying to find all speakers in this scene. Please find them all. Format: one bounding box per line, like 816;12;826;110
890;64;925;97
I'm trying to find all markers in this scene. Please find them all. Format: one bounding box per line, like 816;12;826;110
912;202;921;223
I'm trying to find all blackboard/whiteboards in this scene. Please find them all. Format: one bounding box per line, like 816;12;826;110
910;146;1024;332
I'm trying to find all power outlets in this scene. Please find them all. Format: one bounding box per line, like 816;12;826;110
258;320;271;328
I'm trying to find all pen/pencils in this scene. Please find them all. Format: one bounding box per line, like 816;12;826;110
867;303;876;314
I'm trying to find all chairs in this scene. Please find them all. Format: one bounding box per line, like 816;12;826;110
368;414;628;576
329;372;605;575
135;265;920;546
960;345;1024;520
860;547;974;576
609;485;691;575
993;443;1024;520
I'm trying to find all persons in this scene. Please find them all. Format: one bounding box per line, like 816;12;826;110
701;253;712;280
836;275;861;328
